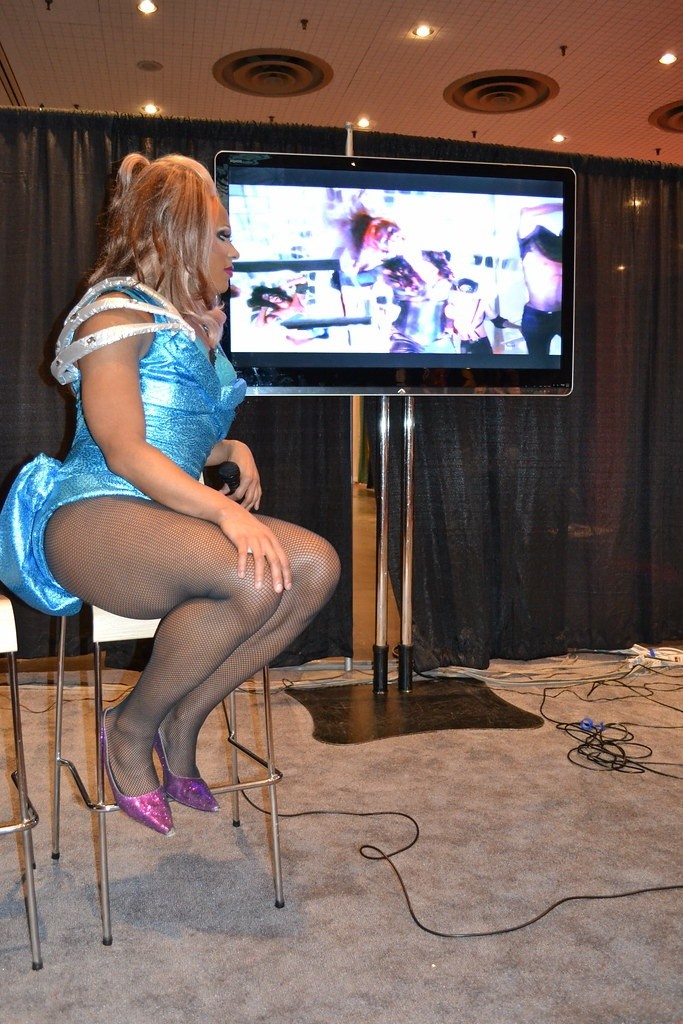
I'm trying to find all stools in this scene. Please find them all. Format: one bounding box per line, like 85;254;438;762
0;579;285;970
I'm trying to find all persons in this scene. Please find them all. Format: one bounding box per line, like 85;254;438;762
0;152;340;840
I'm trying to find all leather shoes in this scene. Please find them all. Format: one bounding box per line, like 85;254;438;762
153;727;221;812
99;706;176;839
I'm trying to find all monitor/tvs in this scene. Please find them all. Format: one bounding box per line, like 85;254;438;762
212;148;578;400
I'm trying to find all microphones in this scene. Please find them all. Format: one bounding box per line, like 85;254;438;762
219;461;251;512
